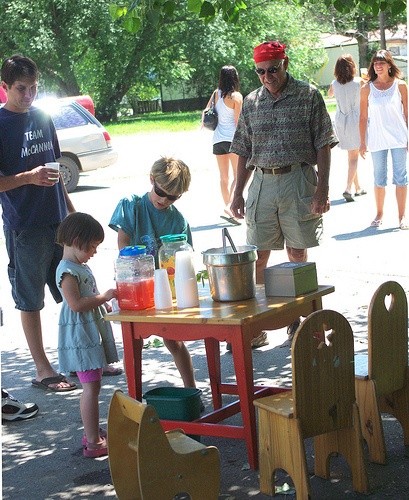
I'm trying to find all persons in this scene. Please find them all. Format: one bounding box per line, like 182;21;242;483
328;54;367;202
0;53;124;392
110;155;206;411
200;64;249;226
55;211;124;458
224;41;340;350
359;49;407;229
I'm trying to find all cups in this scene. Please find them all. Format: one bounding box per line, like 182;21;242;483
174;250;199;308
153;269;173;310
45;162;59;183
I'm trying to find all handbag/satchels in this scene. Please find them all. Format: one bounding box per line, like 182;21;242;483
203;91;217;130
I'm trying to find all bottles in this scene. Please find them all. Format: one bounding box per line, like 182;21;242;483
158;234;193;307
114;245;156;310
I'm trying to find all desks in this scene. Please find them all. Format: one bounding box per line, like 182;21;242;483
103;285;334;470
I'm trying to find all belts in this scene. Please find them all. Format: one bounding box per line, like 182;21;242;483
256;162;309;174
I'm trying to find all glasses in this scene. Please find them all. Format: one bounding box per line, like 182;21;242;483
255;59;284;75
154;180;182;201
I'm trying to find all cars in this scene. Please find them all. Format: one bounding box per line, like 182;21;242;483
0;86;116;193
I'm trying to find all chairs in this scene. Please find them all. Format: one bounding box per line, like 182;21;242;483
333;281;409;465
252;309;372;500
107;389;223;500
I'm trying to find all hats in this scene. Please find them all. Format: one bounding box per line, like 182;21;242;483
255;42;286;63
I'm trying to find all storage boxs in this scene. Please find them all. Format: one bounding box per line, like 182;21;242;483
263;261;317;297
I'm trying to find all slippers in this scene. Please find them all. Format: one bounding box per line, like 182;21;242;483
220;215;242;225
342;191;354;203
32;373;77;391
70;364;123;376
355;190;367;196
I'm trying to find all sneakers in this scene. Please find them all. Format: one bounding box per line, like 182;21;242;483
1;388;39;422
227;333;267;350
288;317;303;341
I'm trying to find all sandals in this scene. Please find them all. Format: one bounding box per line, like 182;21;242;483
398;221;409;230
371;220;383;226
83;428;108;458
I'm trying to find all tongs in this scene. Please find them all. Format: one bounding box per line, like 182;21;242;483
222;228;237;253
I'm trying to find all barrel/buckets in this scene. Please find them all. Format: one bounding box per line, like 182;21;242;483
201;245;259;302
